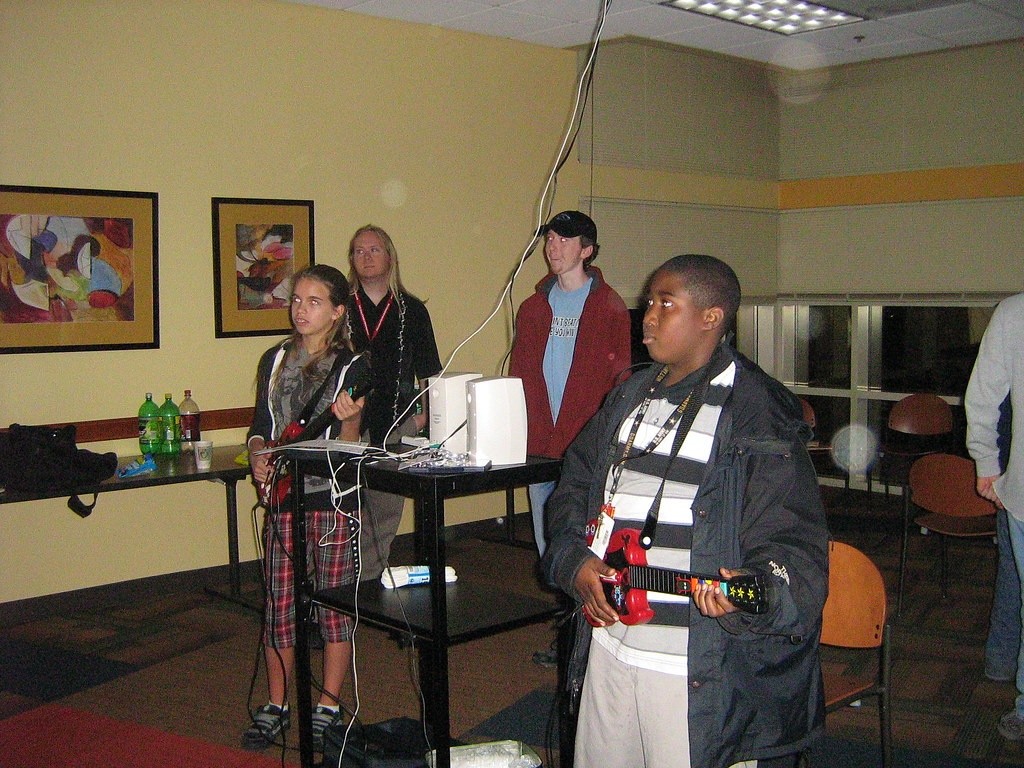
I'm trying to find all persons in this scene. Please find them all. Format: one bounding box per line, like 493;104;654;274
242;263;371;752
507;210;632;673
964;291;1024;741
536;254;830;768
308;224;442;652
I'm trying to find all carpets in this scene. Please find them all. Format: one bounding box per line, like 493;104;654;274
0;705;299;768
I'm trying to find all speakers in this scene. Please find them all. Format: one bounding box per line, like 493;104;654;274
428;372;528;466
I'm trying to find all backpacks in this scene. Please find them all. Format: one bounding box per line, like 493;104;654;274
0;423;119;518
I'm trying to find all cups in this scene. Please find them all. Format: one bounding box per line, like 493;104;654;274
193;441;213;469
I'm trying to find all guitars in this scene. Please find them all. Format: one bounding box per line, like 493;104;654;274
251;374;376;508
581;528;768;626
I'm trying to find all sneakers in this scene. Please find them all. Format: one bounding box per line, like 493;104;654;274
313;704;343;751
241;702;291;749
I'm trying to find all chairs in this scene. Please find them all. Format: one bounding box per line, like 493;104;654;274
799;393;999;620
817;538;893;768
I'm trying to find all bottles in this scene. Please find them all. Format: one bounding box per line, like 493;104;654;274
178;389;201;452
137;393;159;454
159;393;181;454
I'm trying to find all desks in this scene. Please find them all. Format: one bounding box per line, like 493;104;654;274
0;445;268;616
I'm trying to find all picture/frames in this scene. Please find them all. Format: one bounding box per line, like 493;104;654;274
211;197;314;339
0;183;159;355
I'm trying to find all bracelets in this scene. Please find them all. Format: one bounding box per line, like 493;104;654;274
415;415;427;433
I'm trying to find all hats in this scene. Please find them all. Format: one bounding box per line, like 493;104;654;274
534;210;597;243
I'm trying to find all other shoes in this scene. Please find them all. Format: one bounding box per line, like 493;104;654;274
997;709;1024;741
534;646;560;667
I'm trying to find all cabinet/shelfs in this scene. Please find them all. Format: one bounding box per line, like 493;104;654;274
283;442;574;768
